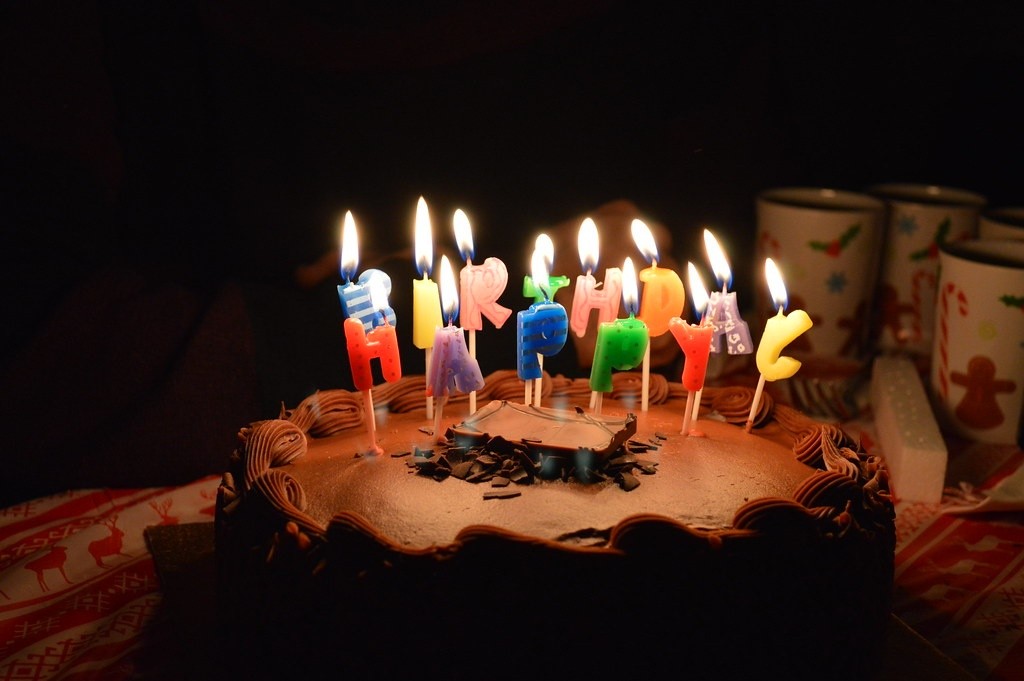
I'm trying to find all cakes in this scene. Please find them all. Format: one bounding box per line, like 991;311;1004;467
214;369;897;681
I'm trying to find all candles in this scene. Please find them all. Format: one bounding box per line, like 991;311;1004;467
745;256;814;433
588;258;648;415
343;274;401;450
452;209;512;414
630;219;685;414
668;260;714;435
336;210;396;432
570;216;622;408
412;194;444;420
516;248;568;407
425;254;485;440
691;228;754;421
522;233;570;408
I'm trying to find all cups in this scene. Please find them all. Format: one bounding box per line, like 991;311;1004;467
757;188;885;396
980;211;1024;239
930;242;1024;446
876;185;989;368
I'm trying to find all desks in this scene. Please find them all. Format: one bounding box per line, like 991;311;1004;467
0;378;1024;681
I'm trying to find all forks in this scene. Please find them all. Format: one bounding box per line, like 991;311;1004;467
789;380;857;419
